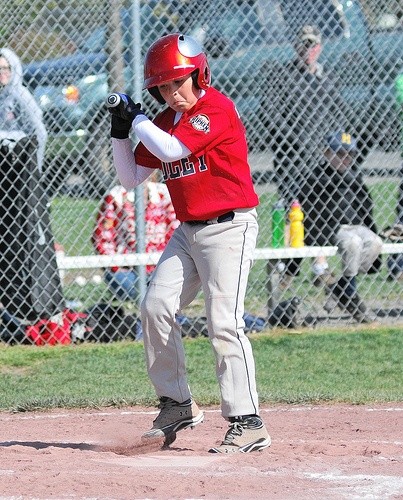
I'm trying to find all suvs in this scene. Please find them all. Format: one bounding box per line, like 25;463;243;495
22;0;206;87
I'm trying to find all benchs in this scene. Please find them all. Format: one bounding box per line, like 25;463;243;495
54;243;403;316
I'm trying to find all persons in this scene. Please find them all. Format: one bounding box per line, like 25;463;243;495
105;33;271;453
0;25;383;455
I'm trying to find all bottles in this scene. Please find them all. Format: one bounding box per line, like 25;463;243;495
288;199;304;248
271;202;286;249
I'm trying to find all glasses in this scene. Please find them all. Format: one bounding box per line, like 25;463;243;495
296;40;320;48
0;66;10;73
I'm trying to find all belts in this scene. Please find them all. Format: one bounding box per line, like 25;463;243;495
184;211;234;225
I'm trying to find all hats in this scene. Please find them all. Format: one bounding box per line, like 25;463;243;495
293;25;323;44
326;133;357;151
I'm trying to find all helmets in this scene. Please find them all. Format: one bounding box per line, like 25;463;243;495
141;34;211;105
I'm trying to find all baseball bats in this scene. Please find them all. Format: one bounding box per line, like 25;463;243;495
104;92;125;113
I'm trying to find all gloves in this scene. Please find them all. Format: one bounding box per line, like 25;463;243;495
110;114;132;139
108;93;145;125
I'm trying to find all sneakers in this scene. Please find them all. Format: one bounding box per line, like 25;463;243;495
278;274;299;292
312;272;337;292
208;415;271;455
325;283;377;324
141;398;204;448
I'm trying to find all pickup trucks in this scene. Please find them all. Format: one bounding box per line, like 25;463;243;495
32;0;403;198
